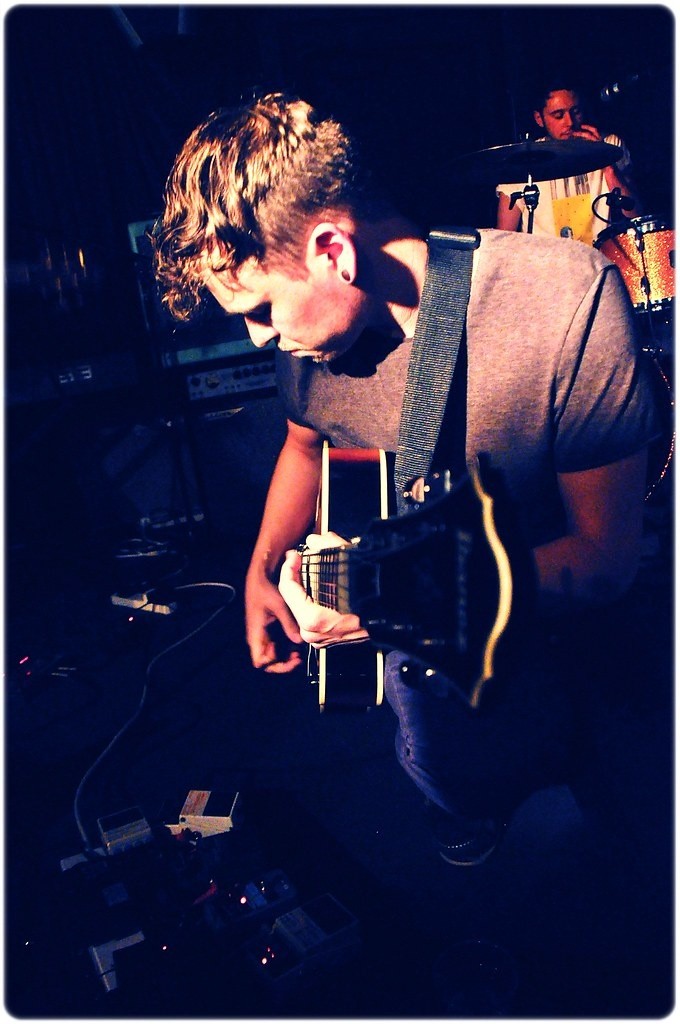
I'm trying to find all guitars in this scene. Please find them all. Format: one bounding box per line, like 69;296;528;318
285;454;515;710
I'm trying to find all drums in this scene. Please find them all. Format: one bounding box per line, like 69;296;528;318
596;208;674;315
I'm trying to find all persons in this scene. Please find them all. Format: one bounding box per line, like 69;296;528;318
492;84;636;251
147;88;666;866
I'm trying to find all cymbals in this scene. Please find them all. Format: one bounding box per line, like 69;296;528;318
469;138;623;184
